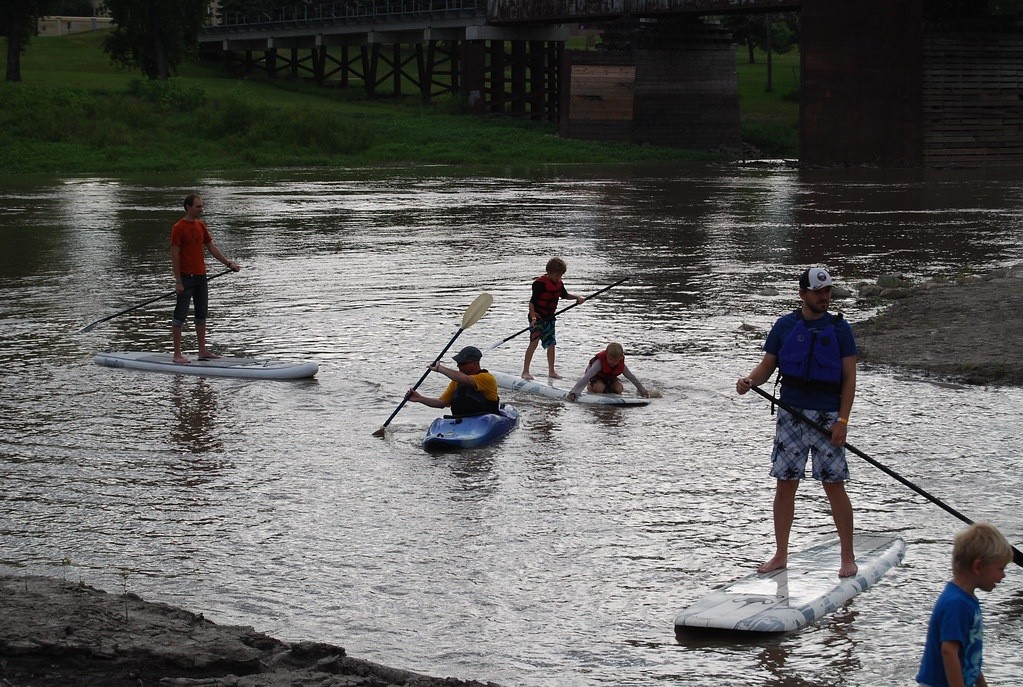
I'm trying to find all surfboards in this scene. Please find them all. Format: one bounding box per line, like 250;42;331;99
489;370;651;407
675;531;904;633
92;349;319;380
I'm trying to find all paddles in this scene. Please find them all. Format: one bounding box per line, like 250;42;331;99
483;275;629;354
742;377;1023;566
80;271;231;333
373;292;495;441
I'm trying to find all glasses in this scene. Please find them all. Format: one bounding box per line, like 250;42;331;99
457;361;474;366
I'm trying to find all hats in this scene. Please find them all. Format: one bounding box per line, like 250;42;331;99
799;268;838;291
452;346;482;364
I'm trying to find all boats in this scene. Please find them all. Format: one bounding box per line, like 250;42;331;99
421;403;520;454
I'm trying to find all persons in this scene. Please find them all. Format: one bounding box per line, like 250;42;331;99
736;267;858;577
565;343;651;402
405;346;502;418
170;194;239;364
915;523;1013;687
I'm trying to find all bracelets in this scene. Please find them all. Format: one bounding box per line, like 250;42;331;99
227;262;231;268
837;418;847;425
521;257;585;382
436;364;440;371
531;317;536;320
